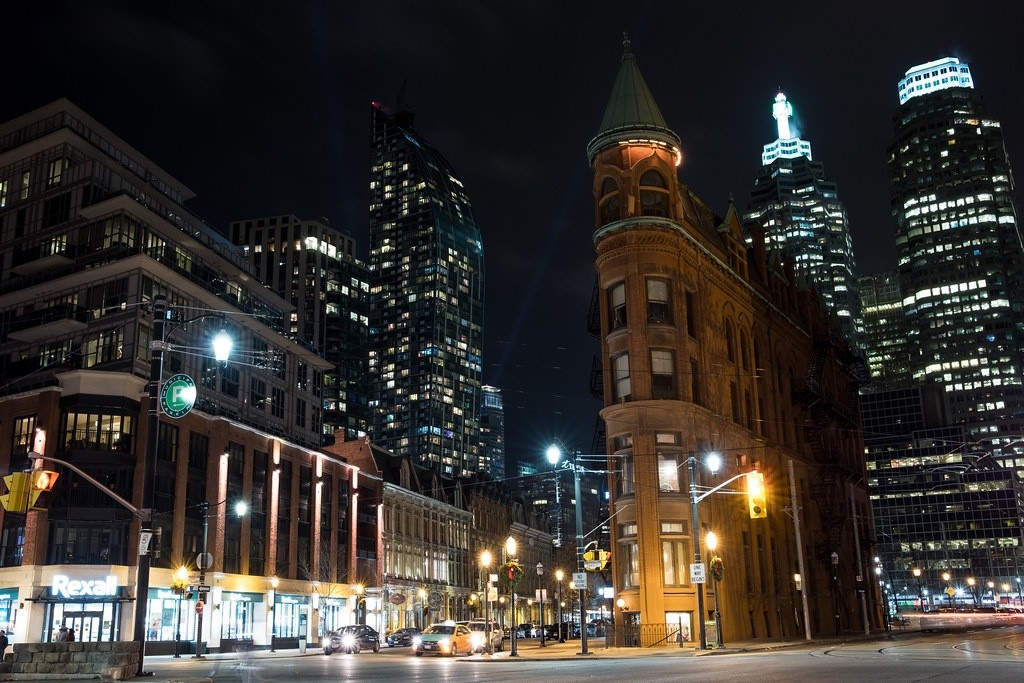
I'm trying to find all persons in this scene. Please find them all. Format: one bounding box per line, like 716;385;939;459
0;630;8;661
67;628;75;641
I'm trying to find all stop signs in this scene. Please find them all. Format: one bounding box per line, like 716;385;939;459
195;601;203;612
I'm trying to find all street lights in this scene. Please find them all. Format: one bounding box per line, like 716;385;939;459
687;450;723;648
875;555;1024;631
546;435;593;656
569;582;575;640
471;593;485;630
419;589;427;631
270;574;279;654
129;293;234;671
480;548;493;656
831;551;840;636
527;598;533;638
499;596;505;638
191;495;247;660
355;583;363;625
536;562;547;648
599;587;605;636
172;564;188;658
506;536;520;658
706;532;726;649
555;569;564;643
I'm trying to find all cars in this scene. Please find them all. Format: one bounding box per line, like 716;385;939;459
413;619;477;657
321;624;380;656
386;627;422;648
452;615;612;653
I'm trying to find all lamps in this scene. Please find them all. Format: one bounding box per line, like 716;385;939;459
617;599;629;611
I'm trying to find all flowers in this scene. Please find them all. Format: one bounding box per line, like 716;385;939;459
710;555;725;581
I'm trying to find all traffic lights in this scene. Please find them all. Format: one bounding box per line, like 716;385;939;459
27;469;60;513
599;551;612;571
746;472;767;519
